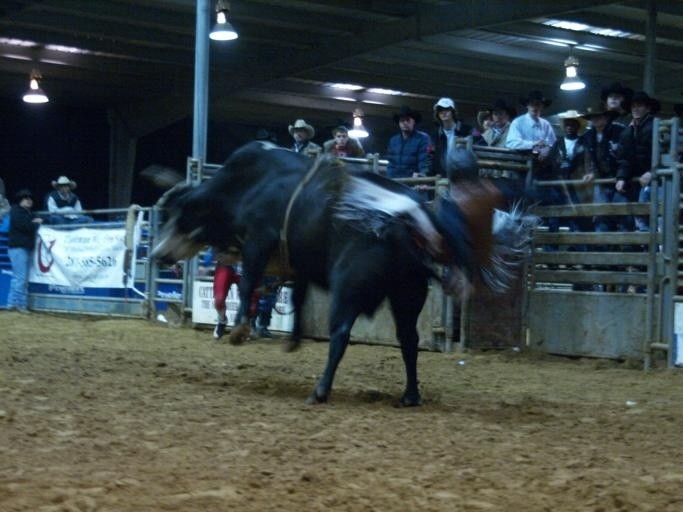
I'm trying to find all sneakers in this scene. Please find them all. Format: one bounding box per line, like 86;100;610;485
213;317;228;340
257;327;276;339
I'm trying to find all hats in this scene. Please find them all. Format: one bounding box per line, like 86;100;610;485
51;175;77;191
476;80;661;137
13;189;35;202
432;98;457;121
392;105;422;124
288;119;314;139
246;131;278;144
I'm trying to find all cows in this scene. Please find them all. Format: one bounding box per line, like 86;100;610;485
140;139;545;409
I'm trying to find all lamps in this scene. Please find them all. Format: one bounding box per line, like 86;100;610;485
346;109;370;140
207;1;241;43
559;44;586;92
19;70;51;105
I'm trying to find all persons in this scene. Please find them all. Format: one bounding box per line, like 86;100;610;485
47;174;94;224
7;189;43;311
